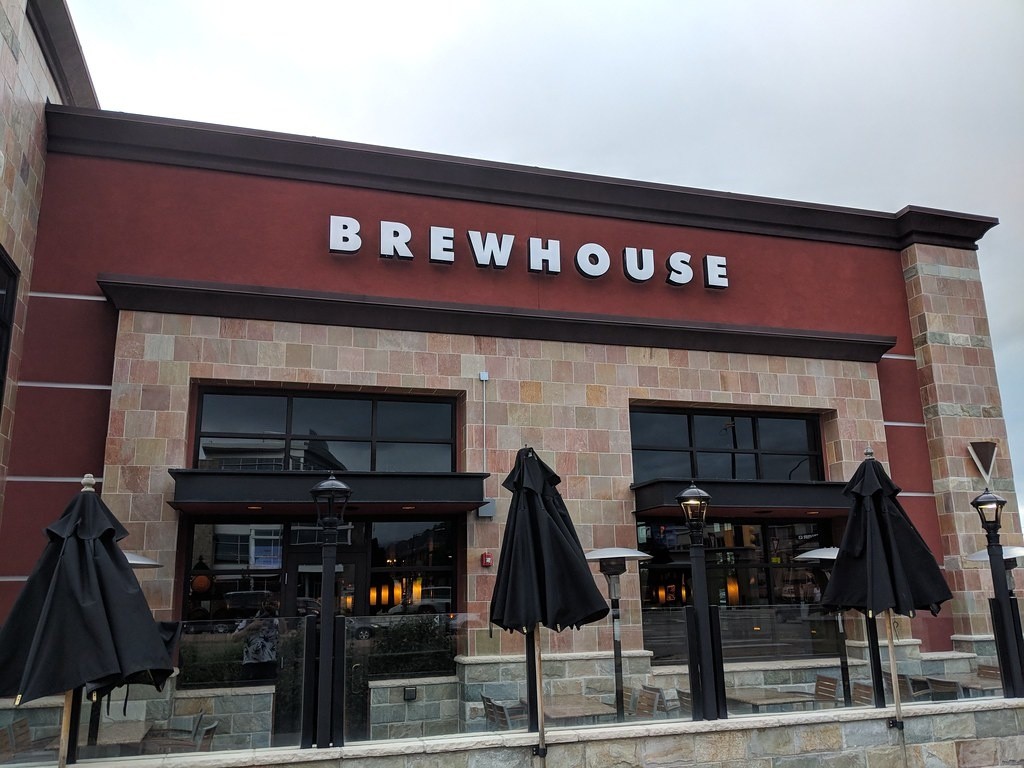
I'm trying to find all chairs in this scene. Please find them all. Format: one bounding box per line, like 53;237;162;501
675;689;693;718
127;720;220;755
926;676;965;700
833;681;874;708
641;684;681;719
882;671;931;702
130;707;206;755
815;674;840;699
10;716;59;760
977;664;1001;680
582;684;636;724
491;701;556;731
598;689;661;726
479;691;527;731
0;726;57;765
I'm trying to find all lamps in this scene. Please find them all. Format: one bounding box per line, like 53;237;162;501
386;558;397;569
584;546;654;576
966;545;1024;591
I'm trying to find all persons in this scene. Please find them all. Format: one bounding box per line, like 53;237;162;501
231;593;287;688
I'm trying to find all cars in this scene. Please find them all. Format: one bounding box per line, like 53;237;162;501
346;618;381;642
208;589;343;628
387;585;452;615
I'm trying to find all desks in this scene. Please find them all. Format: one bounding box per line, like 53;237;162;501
522;695;617;725
908;673;1003;698
725;688;832;713
43;722;154;760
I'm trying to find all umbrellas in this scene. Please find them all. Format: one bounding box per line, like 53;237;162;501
484;444;615;759
0;471;176;766
817;444;954;725
